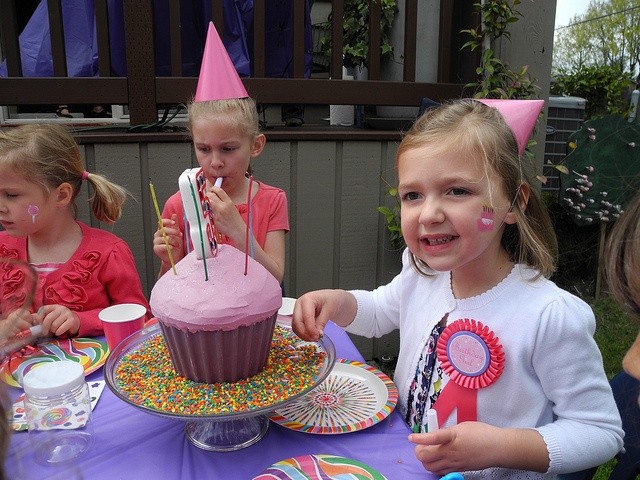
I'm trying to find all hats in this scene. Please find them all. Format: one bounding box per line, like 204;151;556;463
473;99;545;156
193;21;249;102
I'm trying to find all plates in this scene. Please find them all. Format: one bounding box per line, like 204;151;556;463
250;454;387;479
268;356;399;436
2;335;112;390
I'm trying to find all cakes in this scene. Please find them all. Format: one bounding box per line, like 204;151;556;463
150;166;281;381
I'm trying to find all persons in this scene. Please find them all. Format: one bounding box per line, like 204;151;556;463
599;186;639;409
252;103;314;129
1;122;151;352
152;97;290;287
53;104;111;119
291;97;627;479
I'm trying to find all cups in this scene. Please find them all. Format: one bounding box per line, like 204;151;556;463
96;301;148;354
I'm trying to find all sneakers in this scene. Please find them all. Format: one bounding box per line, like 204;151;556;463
91;109;108;118
286;117;301;126
55;105;73;119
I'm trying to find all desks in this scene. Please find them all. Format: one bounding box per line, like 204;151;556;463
0;300;441;480
